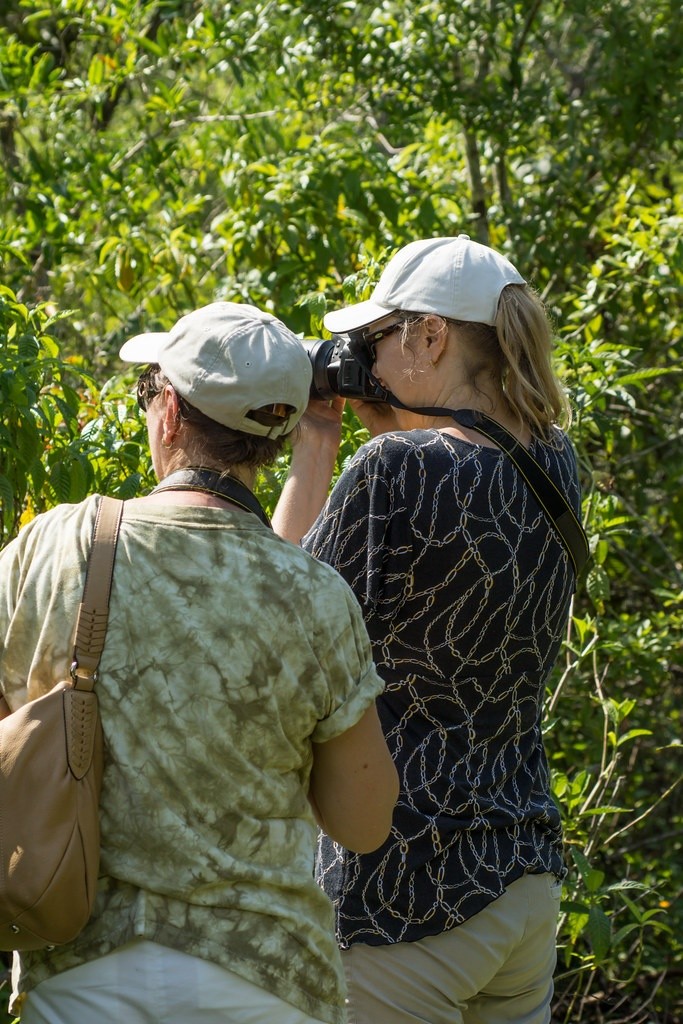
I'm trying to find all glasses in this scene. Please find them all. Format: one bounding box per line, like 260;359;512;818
362;316;464;360
137;379;191;420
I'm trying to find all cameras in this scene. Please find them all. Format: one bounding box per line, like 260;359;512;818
298;338;397;404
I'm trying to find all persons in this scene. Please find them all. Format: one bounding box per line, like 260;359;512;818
268;236;583;1024
0;301;398;1024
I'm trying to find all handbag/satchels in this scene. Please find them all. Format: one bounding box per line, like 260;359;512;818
0;496;126;951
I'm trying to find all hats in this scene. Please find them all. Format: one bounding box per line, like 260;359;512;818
119;301;312;440
323;233;527;334
